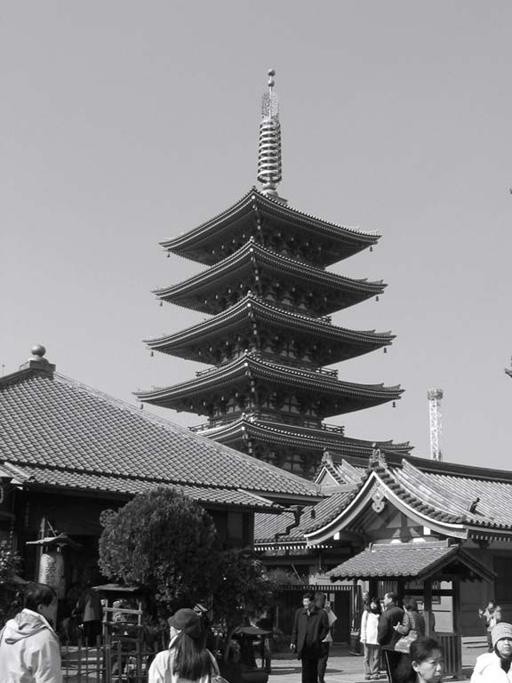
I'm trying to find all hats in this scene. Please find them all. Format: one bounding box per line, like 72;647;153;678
167;607;202;639
490;621;512;650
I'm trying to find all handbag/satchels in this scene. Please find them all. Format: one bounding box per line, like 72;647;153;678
393;630;418;654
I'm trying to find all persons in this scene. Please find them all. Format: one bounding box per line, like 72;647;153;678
360;597;382;680
0;583;62;683
425;636;426;637
148;608;227;683
378;592;425;683
470;622;512;683
410;637;444;683
290;594;332;682
479;601;501;652
